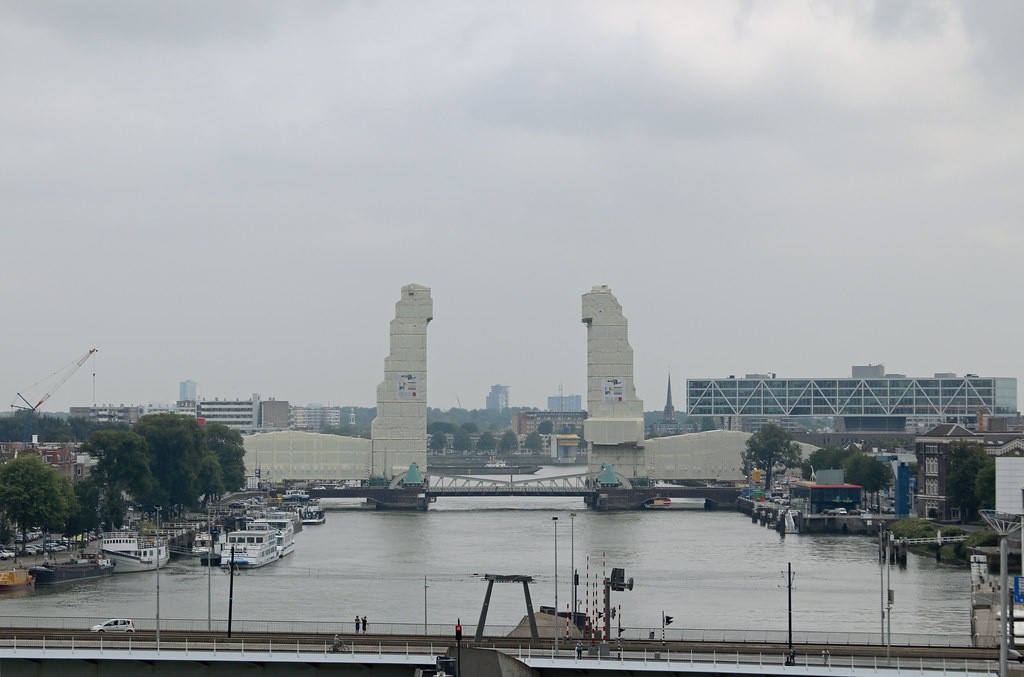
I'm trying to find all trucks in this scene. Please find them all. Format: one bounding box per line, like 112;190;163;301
835;507;847;515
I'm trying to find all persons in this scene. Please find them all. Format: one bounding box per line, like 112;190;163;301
333;634;342;652
790;645;795;663
354;616;361;633
821;648;830;667
11;551;84;567
361;616;367;634
576;640;584;660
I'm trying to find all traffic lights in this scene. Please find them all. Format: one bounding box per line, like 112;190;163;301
666;616;673;625
456;625;462;640
619;628;625;637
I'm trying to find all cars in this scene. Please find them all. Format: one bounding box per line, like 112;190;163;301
849;510;857;515
869;504;895;514
765;493;790;504
824;509;829;513
229;496;263;508
91;619;135;633
827;510;836;516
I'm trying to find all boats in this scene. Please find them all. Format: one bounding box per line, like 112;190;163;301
192;533;212;557
302;498;326;525
30;547;117;585
0;567;33;591
253;518;296;557
98;516;170;573
219;530;281;569
427;464;543;474
